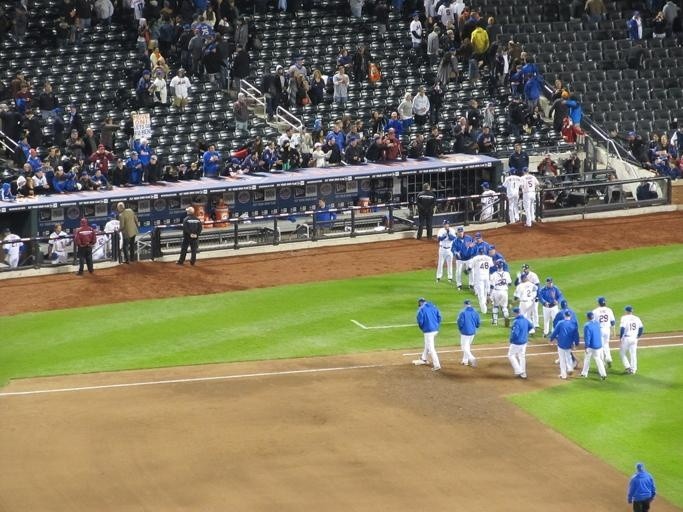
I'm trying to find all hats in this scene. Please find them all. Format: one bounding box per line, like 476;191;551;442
417;297;425;302
564;309;571;315
520;271;529;280
521;167;529;172
141;69;151;75
480;182;489;188
624;305;632;312
508;167;516;174
585;312;593;318
70;129;79;133
512;307;520;312
456;227;463;232
80;218;89;226
185;207;194;213
477;246;486;252
560;300;567;308
636;463;644;470
546;277;553;283
129;151;138;156
475;232;481;238
488;245;495;250
597;297;605;304
464;299;471;305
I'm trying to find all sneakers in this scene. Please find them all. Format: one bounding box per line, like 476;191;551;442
436;278;440;283
554;358;559;364
519;372;527;378
504;317;510;327
512;372;523;377
567;370;573;376
573;359;580;369
607;362;611;368
621;368;633;374
533;323;539;328
456;286;463;290
468;285;474;290
523;224;531;228
459;361;468;365
419;357;426;363
600;375;606;381
447;279;452;283
471;358;476;367
542;333;548;338
430;367;441;371
577;374;586;377
508;221;515;224
531;220;536;224
528;329;535;334
515;218;519;223
559;375;566;379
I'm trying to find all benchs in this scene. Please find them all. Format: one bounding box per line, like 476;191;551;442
39;205;437;256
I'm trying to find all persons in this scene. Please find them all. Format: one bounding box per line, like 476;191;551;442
412;217;644;380
0;3;682;275
626;462;657;512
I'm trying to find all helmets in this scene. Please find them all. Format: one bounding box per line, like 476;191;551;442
495;259;505;268
520;263;529;270
443;219;449;225
